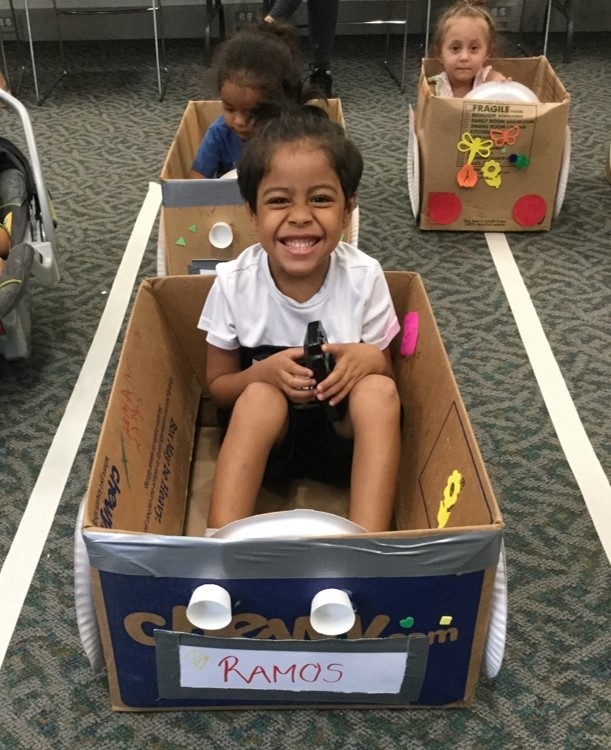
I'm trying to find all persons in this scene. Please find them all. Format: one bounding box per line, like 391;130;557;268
257;0;340;99
195;103;402;540
428;0;512;101
185;21;329;183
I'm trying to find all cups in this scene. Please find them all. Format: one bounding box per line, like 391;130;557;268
208;221;234;250
186;584;232;631
310;589;356;636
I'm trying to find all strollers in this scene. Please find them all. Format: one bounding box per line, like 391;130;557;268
0;87;62;374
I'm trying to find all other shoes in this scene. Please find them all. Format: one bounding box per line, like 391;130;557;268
304;62;334;100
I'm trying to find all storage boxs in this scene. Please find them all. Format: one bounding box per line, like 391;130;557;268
73;270;507;713
406;54;570;233
156;96;360;275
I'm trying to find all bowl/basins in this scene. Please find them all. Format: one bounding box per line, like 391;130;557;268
464;81;538;104
